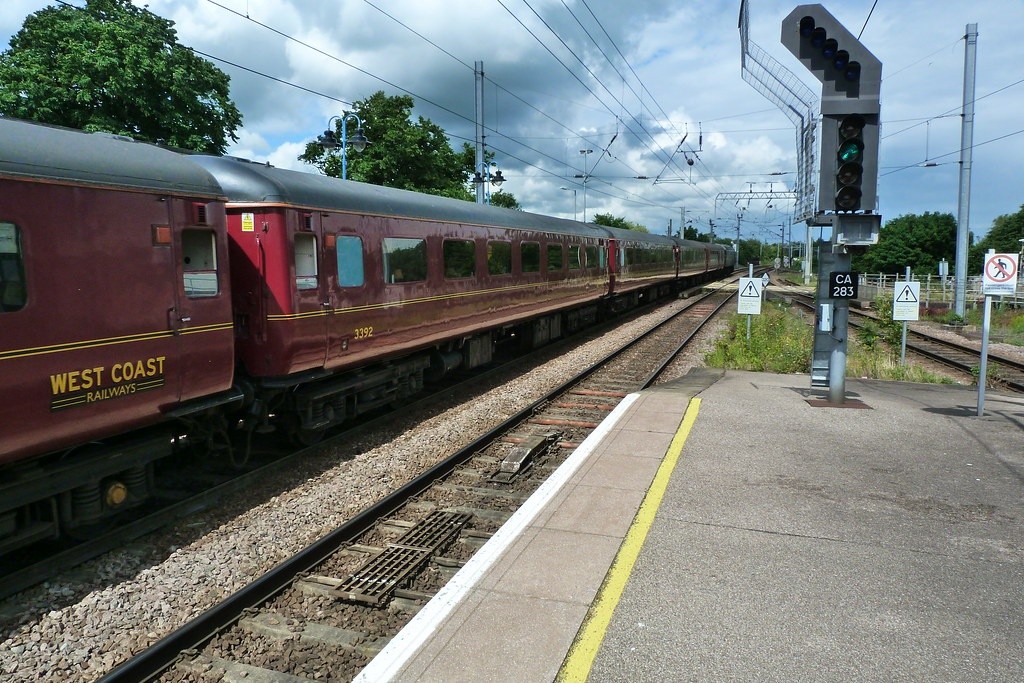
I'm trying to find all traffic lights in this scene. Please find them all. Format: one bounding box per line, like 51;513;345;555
834;113;865;213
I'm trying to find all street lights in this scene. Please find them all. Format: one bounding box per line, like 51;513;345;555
316;114;372;180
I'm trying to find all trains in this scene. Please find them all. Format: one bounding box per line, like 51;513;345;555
1;116;738;561
